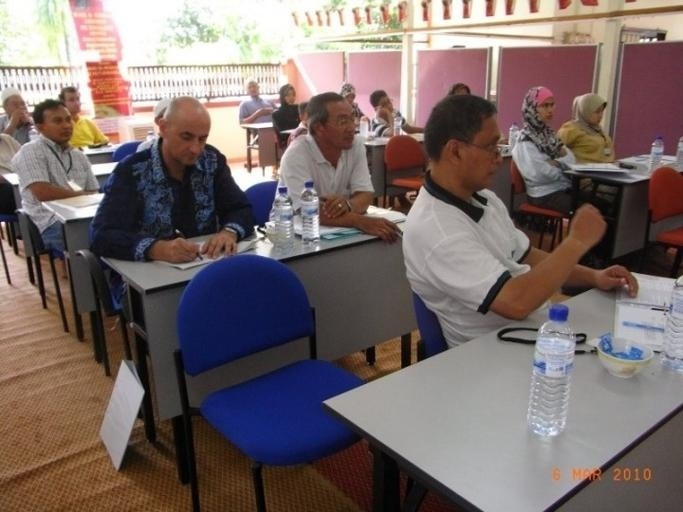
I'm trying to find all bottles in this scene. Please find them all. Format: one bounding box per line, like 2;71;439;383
146;131;154;140
673;134;683;175
659;275;683;376
526;303;575;440
297;178;321;245
274;186;298;256
393;111;403;137
648;134;665;179
508;122;520;152
358;116;373;141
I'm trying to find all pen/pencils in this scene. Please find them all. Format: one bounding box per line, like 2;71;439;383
623;321;665;332
175;229;204;260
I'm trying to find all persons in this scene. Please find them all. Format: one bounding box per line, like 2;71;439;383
135;97;171;153
240;80;424;244
0;88;34;144
399;94;640;350
0;134;23;215
9;99;102;262
86;95;256;324
448;84;617;270
57;87;109;151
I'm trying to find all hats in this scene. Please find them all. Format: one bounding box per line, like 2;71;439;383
153;98;170;125
1;88;19;107
341;83;356;98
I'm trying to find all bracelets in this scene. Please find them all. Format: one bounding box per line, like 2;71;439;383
223;225;236;234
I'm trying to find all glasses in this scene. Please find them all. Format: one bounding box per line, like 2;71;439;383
325;117;354;128
444;136;503;154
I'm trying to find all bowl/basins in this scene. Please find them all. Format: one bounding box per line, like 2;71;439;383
598;334;654;379
